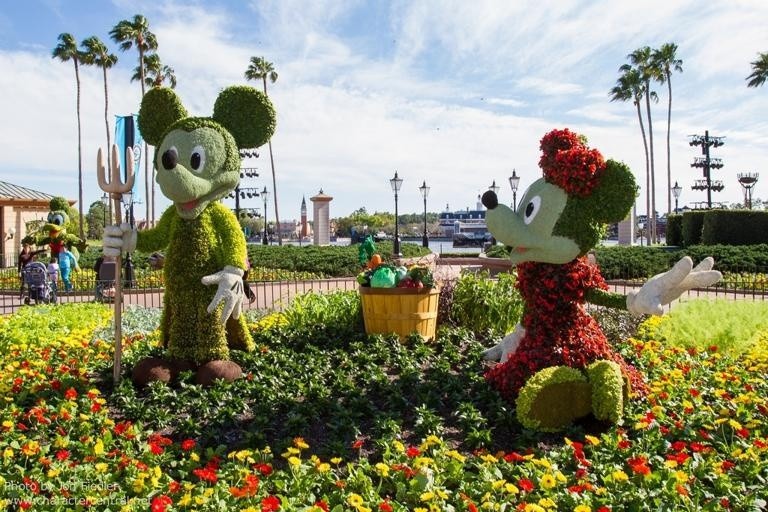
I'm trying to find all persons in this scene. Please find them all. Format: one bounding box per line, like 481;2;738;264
46;246;79;295
18;243;47;299
242;259;256;303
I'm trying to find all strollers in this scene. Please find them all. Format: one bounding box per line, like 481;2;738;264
23;262;57;304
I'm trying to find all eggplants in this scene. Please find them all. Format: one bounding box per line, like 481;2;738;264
357;272;372;285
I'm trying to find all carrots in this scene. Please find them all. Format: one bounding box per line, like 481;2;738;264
358;235;381;268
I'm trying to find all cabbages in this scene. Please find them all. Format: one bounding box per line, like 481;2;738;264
371;266;408;288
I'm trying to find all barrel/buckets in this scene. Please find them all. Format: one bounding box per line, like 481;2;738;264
358;285;441;340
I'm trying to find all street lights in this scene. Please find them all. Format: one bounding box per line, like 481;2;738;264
489;180;500;247
296;222;304;247
638;219;644;246
509;169;521;213
671;181;682;215
101;192;108;228
364;224;368;240
390;171;403;259
122;190;133;289
419;180;430;248
260;185;274;246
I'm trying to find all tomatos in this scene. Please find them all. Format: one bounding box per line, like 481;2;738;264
398;277;424;290
